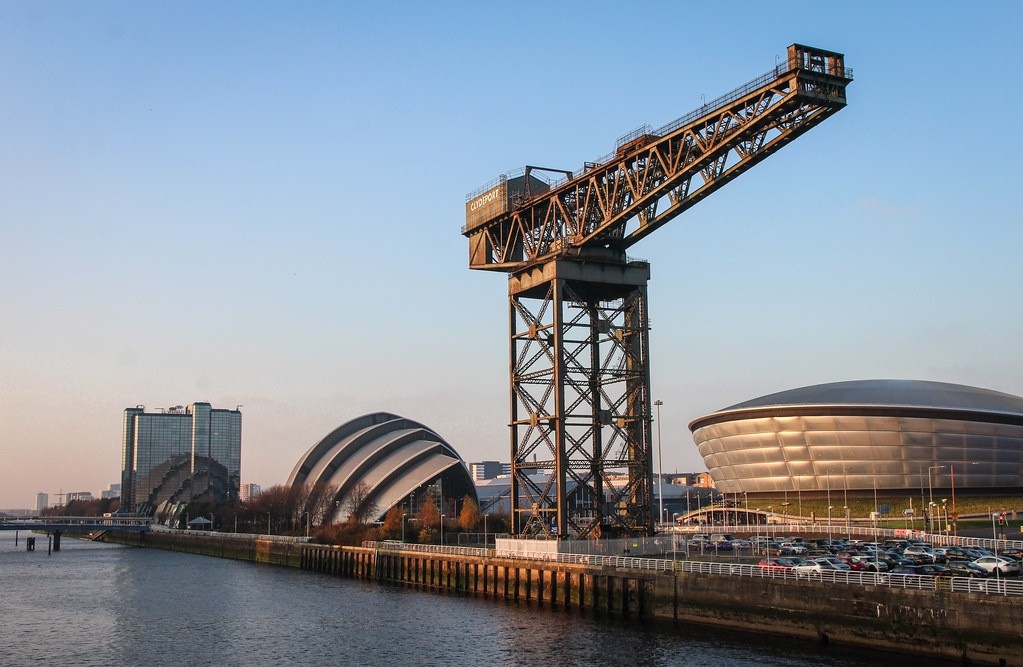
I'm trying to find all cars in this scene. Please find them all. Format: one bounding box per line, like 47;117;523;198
681;533;1021;576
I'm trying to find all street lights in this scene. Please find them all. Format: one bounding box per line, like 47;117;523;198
769;506;775;539
991;511;1003;593
267;511;271;535
663;508;670;535
185;512;189;532
672;512;679;562
828;505;835;547
654;399;664;528
401;513;407;543
928;465;947;530
305;511;310;537
440;514;446;546
234;512;237;533
871;511;882;585
484;513;489;549
209;512;215;531
765;513;773;576
756;508;760;556
844;506;851;541
402;482;557;516
928;497;950;565
